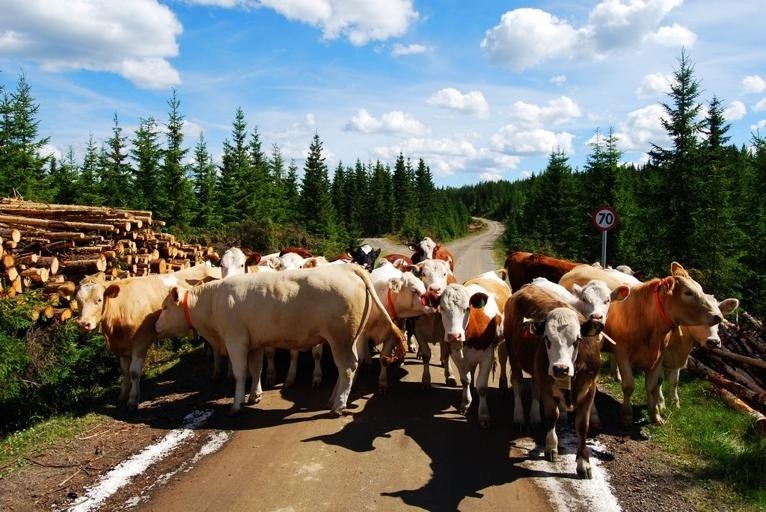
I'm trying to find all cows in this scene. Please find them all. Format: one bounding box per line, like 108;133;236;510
60;266;218;409
172;235;739;479
157;263;411;420
